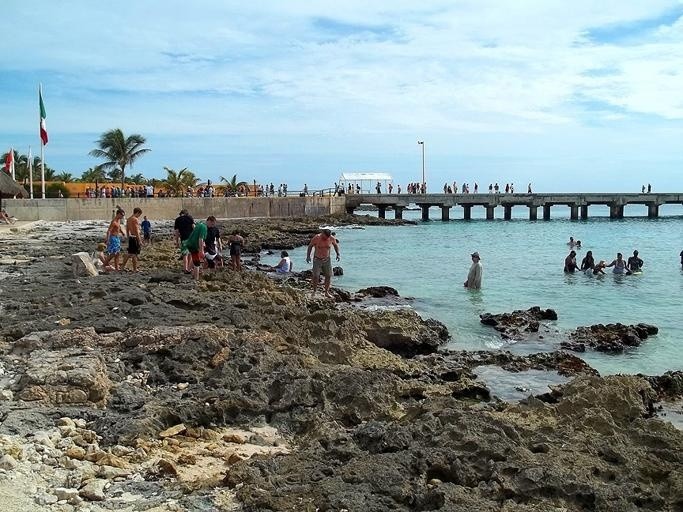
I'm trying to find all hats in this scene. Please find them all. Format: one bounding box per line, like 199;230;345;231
471;251;481;260
95;242;107;251
633;250;639;254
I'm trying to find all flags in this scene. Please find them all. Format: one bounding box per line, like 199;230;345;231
39;88;49;145
27;149;31;168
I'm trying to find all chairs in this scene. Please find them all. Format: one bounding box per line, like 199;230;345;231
1;208;15;225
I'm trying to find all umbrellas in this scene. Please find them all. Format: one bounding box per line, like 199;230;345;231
0;170;29;211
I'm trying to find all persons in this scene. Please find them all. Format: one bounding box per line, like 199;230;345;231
564;236;644;276
5;152;12;173
85;182;287;198
679;250;683;271
641;185;645;193
0;209;10;224
307;229;340;298
303;183;309;196
333;182;514;197
103;205;245;282
257;251;293;273
528;183;532;193
464;251;482;290
648;183;651;193
